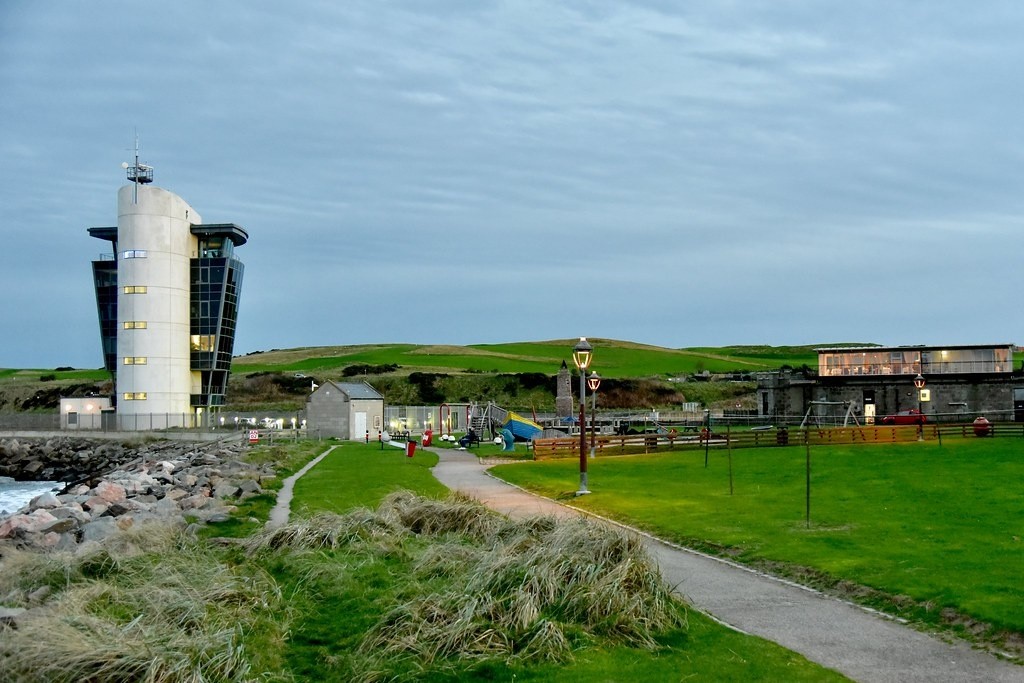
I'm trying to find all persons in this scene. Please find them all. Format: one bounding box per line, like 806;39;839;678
454;428;475;449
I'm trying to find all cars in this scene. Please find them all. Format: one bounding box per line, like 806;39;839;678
384;416;419;430
293;373;305;378
235;418;257;425
260;418;277;427
878;408;926;424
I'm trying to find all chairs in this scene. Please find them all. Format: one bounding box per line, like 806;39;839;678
862;367;869;374
848;367;858;375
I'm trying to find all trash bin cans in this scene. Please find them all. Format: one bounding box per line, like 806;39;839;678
407;439;419;457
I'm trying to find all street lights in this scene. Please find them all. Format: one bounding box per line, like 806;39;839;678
914;373;925;441
586;371;602;459
572;337;593;497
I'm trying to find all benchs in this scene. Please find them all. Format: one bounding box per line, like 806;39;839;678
467;435;479;448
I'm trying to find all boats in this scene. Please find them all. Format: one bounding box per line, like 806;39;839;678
502;411;544;441
751;425;773;431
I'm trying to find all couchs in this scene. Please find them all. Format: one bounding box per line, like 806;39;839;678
831;369;842;375
883;367;891;374
